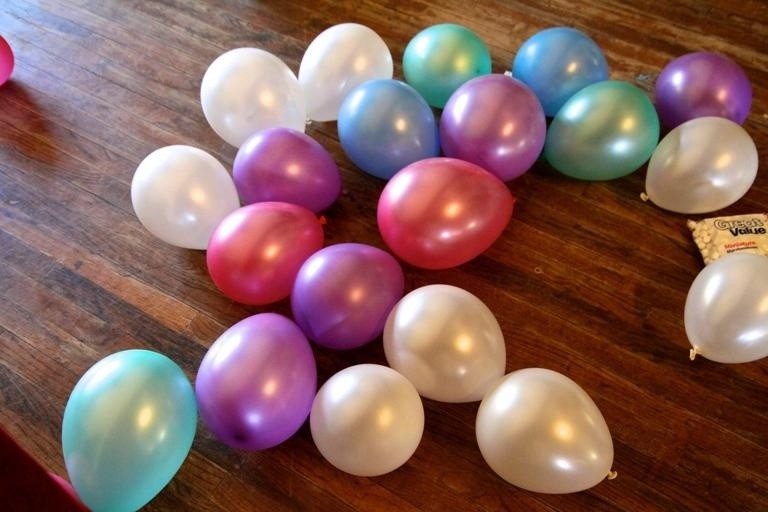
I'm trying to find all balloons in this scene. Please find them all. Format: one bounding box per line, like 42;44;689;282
0;35;14;87
59;21;763;510
